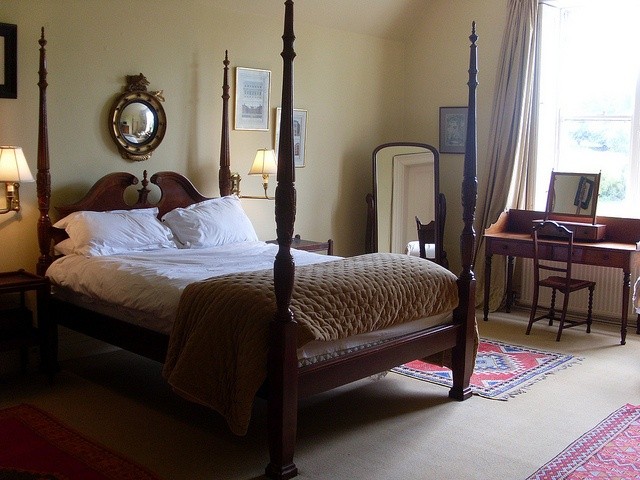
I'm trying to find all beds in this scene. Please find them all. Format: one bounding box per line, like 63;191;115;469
36;0;481;480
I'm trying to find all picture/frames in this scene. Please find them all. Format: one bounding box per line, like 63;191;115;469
439;107;469;154
0;22;17;99
574;176;594;209
235;67;272;132
275;107;309;169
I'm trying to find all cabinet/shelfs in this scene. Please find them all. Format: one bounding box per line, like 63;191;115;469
531;219;607;242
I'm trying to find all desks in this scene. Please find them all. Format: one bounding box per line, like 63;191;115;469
483;209;640;345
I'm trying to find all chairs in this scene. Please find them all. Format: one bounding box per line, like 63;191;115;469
526;220;596;341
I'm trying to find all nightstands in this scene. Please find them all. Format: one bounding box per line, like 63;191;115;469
0;269;50;382
265;235;333;255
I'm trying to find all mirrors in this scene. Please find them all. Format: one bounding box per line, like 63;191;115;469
366;142;446;268
108;72;167;160
545;169;601;224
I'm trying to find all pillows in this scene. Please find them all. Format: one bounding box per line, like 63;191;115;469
52;207;177;258
52;238;162;258
161;194;259;248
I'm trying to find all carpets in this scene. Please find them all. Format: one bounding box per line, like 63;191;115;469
0;402;164;480
526;403;639;479
389;337;585;400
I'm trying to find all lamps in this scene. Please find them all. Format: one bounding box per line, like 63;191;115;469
0;146;35;214
231;148;278;200
